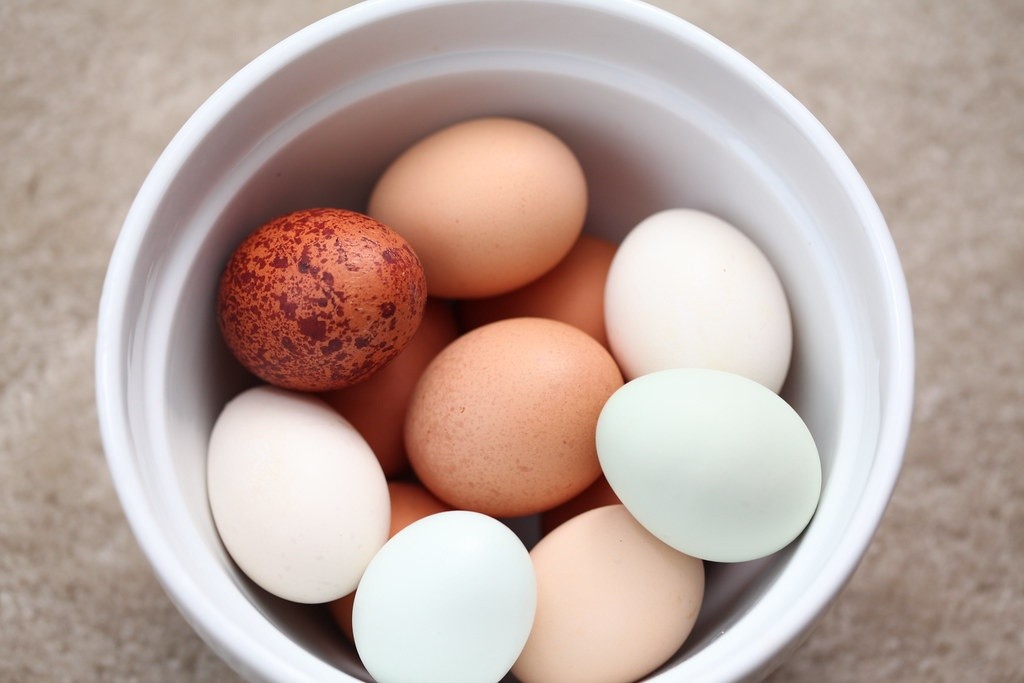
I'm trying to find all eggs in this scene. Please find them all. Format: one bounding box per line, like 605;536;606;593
206;118;821;683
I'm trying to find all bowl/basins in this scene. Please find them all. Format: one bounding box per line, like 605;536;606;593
94;1;916;683
